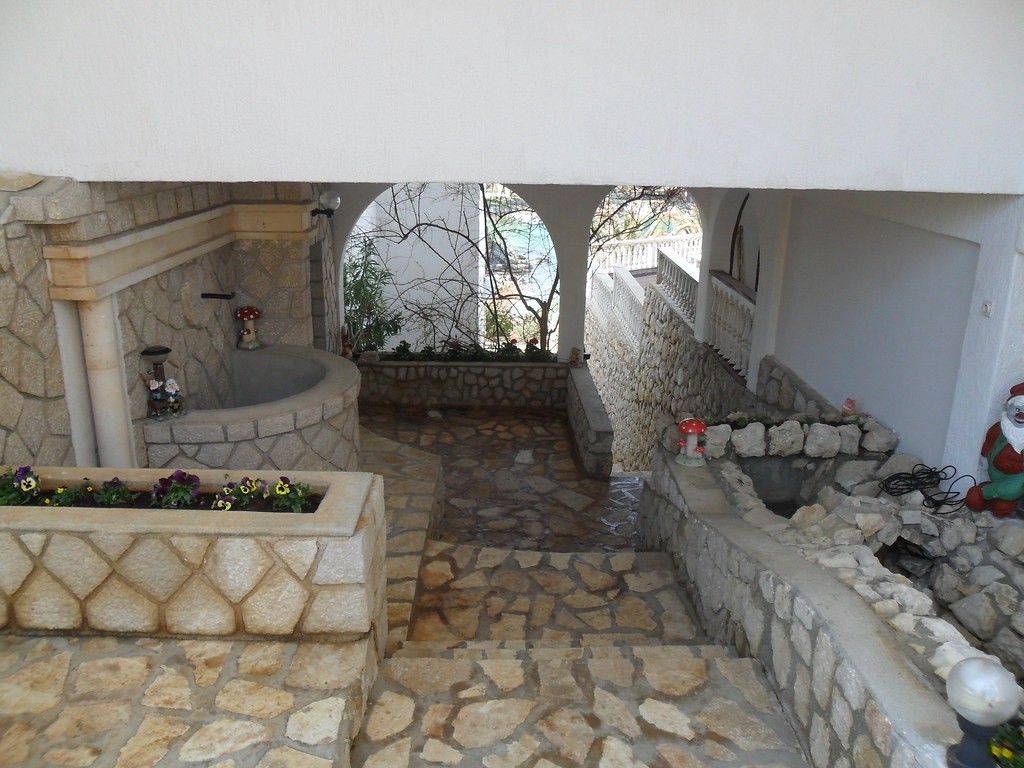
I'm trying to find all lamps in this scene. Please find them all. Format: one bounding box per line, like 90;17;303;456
311;190;342;218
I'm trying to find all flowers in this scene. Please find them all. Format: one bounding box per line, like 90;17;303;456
0;465;311;514
393;336;557;362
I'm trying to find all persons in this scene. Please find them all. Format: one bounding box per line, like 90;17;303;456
965;382;1024;519
165;378;186;416
149;379;163;417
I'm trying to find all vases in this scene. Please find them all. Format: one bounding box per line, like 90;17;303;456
0;465;387;639
350;350;563;408
